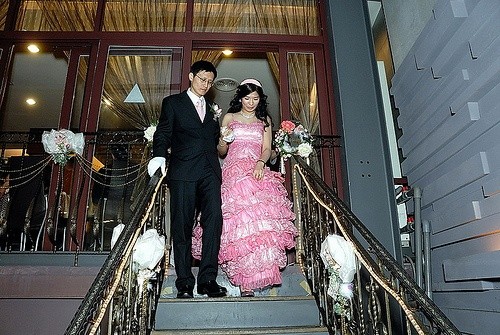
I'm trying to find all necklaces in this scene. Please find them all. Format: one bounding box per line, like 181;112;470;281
241;110;255;118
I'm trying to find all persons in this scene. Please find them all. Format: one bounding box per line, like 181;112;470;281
192;77;299;298
147;60;228;299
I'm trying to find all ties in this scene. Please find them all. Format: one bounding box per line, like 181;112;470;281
196;99;205;123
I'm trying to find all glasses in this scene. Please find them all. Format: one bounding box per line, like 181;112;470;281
195;74;214;87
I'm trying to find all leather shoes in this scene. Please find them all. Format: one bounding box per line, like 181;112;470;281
197;280;228;296
176;285;194;298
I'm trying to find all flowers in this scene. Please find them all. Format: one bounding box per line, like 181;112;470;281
141;115;159;148
209;102;223;120
273;120;315;172
52;134;71;165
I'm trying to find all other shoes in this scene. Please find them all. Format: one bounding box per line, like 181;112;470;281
238;285;255;297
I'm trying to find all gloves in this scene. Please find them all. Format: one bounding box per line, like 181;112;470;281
222;131;235;143
147;157;167;178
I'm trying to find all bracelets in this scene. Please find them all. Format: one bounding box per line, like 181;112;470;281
257;160;265;167
